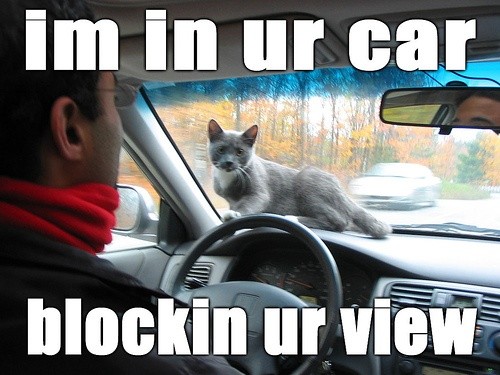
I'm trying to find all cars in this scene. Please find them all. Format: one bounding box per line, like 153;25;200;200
349;161;441;209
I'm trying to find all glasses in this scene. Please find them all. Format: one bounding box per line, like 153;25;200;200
95;83;135;107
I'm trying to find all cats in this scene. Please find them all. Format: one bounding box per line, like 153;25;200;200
206;119;393;239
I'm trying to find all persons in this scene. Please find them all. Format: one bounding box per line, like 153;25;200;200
448;89;500;127
1;0;247;374
